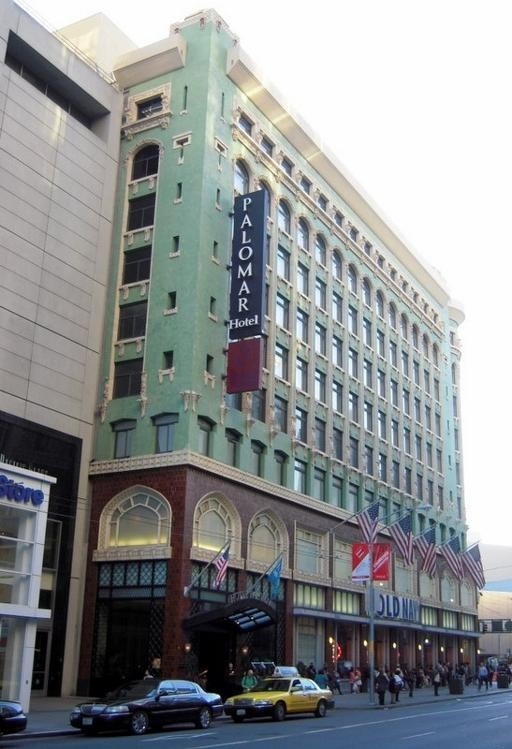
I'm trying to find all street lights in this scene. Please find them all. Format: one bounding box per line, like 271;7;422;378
365;503;434;707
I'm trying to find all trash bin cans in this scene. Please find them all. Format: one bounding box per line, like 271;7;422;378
497;672;509;689
449;674;464;694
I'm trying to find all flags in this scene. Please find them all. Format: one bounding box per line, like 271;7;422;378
438;531;465;582
462;543;486;591
355;500;380;544
212;542;231;593
386;512;414;567
414;527;436;578
266;557;283;601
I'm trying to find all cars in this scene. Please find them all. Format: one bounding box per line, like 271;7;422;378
223;665;337;723
0;699;27;739
68;677;225;736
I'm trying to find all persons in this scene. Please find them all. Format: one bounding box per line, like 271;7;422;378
240;669;258;692
296;653;512;706
144;657;163;681
